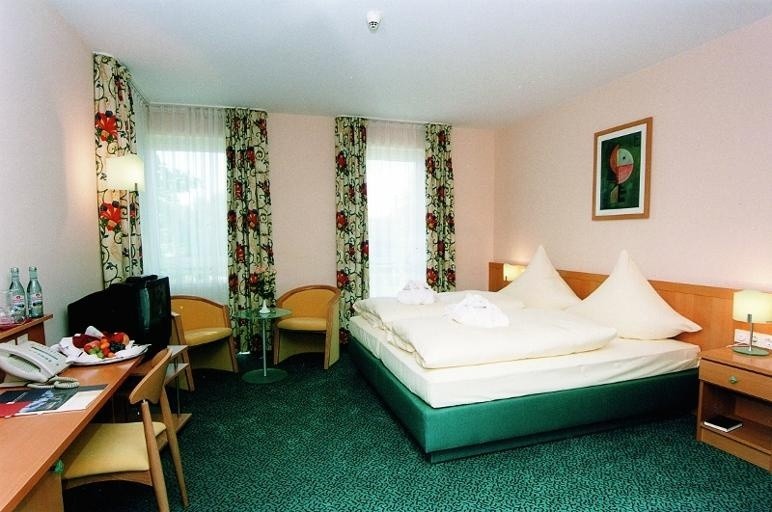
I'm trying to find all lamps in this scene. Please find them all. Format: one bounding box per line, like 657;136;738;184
503;263;525;281
106;155;145;275
727;284;772;356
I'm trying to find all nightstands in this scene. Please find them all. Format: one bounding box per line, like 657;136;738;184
694;342;770;474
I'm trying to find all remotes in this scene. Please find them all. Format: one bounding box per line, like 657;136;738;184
126;275;158;282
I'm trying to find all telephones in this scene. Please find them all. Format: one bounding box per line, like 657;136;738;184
0;340;71;382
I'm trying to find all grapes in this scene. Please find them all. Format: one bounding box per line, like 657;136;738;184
110;343;125;352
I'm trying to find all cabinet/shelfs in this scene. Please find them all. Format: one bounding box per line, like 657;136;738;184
109;344;192;452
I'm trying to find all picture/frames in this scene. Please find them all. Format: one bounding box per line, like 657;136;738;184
592;113;654;222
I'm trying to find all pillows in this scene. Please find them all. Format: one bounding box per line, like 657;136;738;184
490;246;579;311
565;251;702;340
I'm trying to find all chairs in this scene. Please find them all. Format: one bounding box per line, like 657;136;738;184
170;297;238;387
276;282;344;367
61;348;189;512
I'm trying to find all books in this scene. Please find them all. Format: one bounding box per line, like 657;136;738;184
701;412;744;432
0;383;110;417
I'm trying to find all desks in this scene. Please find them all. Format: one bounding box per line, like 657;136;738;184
233;303;291;383
0;308;148;512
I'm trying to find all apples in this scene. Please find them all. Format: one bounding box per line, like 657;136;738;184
113;332;129;347
72;333;88;347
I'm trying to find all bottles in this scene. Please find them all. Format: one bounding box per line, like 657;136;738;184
6;264;44;322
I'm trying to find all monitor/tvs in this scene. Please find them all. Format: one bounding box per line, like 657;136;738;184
68;276;171;364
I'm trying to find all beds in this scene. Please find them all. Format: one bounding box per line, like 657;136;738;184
346;259;702;464
